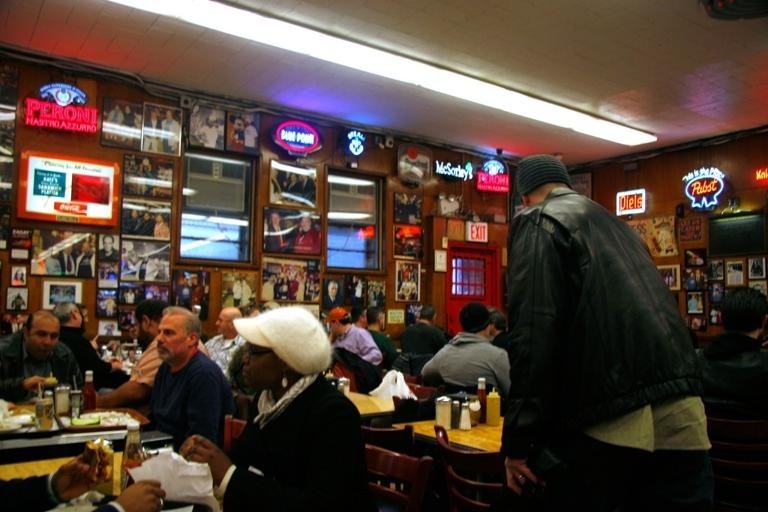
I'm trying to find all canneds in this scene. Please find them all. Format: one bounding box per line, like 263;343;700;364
35;398;56;430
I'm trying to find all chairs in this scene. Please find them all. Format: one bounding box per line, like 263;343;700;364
420;370;494;398
433;423;507;509
398;380;435;406
700;415;767;511
223;415;247;450
358;422;414;454
363;443;435;509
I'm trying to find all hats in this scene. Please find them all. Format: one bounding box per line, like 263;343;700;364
325;307;348;322
517;155;573;194
460;302;492;333
233;306;332;376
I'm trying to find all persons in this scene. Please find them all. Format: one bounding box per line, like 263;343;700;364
697;285;768;506
2;300;510;510
0;93;463;342
666;260;763;329
499;155;719;512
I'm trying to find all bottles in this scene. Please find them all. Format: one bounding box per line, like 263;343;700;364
457;403;472;430
99;342;144;366
476;376;487;425
434;396;452;430
119;418;144;489
36;369;96;429
325;369;350;396
452;400;459;428
486;387;501;426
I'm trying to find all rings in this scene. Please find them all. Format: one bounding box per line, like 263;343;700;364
518;473;526;481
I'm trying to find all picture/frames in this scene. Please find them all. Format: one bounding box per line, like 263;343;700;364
397;142;433;186
98;321;118;336
100;100;141;153
656;246;767;333
394;191;421;223
186;101;226;154
260;253;323;303
96;290;119;319
120;198;172;238
140;103;183;158
96;233;121;260
144;284;170;305
41;278;83;311
119;284;145;307
9;265;26;286
395;260;422;302
322;272;386;331
264;304;320;326
115;311;141;331
169;269;210;322
268;158;319;210
120;238;172;285
405;303;424;327
96;261;119;289
262;205;322;256
8;227;31;262
121;153;174;198
224;110;261;157
31;230;95;279
5;286;29;311
220;270;262;316
0;99;17;157
392;224;424;258
